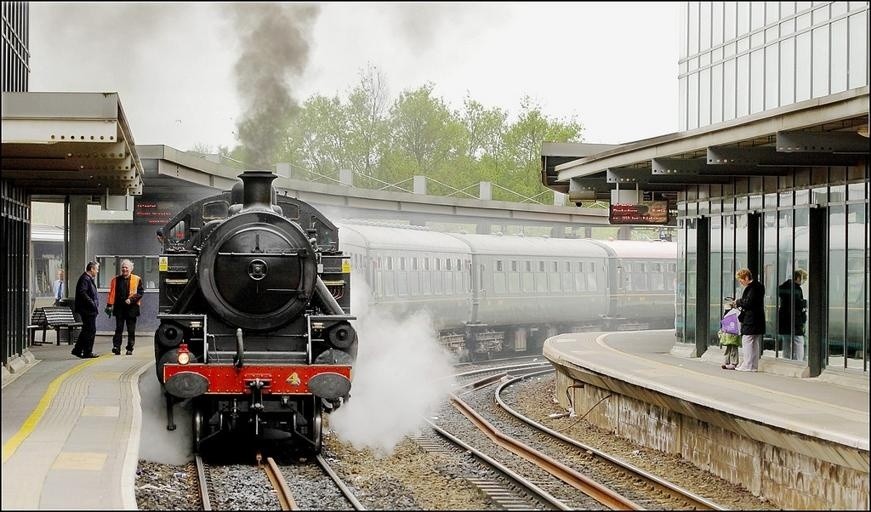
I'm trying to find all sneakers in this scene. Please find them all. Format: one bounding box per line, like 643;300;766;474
112;346;120;355
71;349;100;358
126;350;133;355
721;362;759;372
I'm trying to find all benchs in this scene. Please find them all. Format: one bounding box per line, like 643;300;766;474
29;307;83;346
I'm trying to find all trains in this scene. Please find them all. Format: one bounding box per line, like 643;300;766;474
154;170;870;462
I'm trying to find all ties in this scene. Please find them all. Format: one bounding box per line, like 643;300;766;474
57;282;62;299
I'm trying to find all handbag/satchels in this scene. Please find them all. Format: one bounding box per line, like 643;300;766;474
720;307;742;336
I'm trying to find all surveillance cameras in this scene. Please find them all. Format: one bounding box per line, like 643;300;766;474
576;201;583;208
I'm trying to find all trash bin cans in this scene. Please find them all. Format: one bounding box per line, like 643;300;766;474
58;298;83;343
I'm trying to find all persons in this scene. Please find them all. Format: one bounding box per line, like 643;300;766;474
105;258;144;355
70;261;100;358
721;302;743;370
52;269;65;305
731;269;766;373
778;269;808;360
